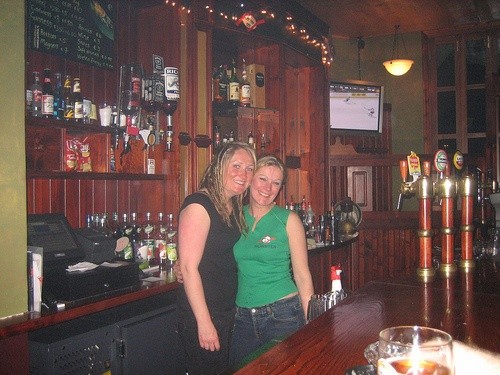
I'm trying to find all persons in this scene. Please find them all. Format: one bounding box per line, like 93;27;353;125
369;108;375;116
173;155;315;365
346;96;350;101
179;141;257;375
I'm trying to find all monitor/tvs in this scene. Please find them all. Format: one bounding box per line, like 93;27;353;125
329;78;384;135
26;213;85;261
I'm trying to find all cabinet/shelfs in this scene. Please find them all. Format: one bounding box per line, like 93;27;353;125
0;0;359;338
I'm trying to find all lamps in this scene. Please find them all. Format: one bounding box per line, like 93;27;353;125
382;25;414;76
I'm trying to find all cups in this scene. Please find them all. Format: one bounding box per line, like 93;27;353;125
306;289;350;326
363;326;457;375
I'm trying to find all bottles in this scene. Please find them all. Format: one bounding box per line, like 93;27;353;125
85;212;177;271
283;193;339;248
111;51;179;149
211;122;265;163
211;54;251;108
25;60;99;124
